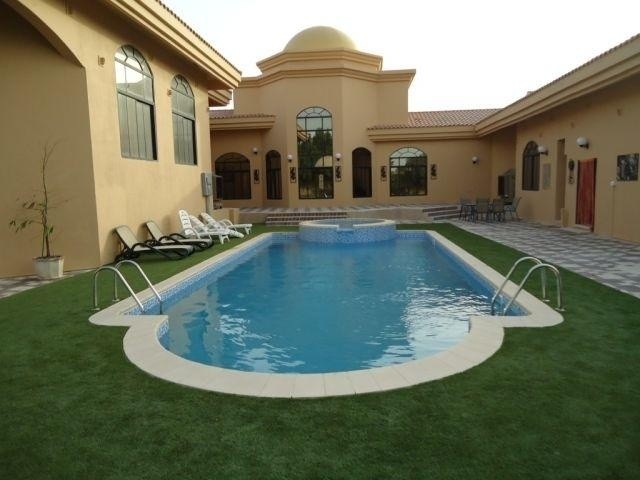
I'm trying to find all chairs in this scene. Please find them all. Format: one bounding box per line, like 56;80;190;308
114;208;252;262
458;195;522;223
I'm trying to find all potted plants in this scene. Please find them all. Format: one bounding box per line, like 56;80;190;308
9;140;70;278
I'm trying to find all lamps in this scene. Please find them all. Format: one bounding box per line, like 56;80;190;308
287;154;293;162
538;146;549;155
472;156;478;163
577;136;589;149
335;153;342;161
253;147;258;154
